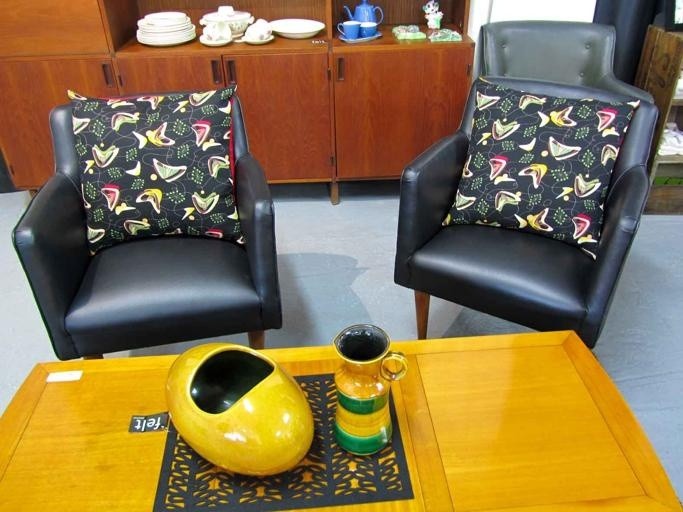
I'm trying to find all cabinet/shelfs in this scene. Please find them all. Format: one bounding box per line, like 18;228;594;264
115;26;335;187
333;22;476;184
636;25;683;214
0;0;123;203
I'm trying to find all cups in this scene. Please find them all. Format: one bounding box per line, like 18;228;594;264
201;22;230;41
333;325;407;457
245;19;271;40
360;20;377;36
338;19;360;41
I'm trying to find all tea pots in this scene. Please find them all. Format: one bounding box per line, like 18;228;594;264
342;1;383;28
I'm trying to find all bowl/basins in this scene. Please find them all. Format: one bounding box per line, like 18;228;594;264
197;6;252;37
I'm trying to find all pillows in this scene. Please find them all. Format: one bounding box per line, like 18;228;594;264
442;78;639;259
66;83;247;258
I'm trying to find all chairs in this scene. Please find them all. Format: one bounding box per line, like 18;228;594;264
476;19;654;107
394;76;659;349
9;82;281;363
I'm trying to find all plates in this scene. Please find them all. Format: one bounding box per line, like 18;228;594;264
199;35;233;46
270;18;324;39
338;32;383;42
242;33;275;45
133;12;197;46
